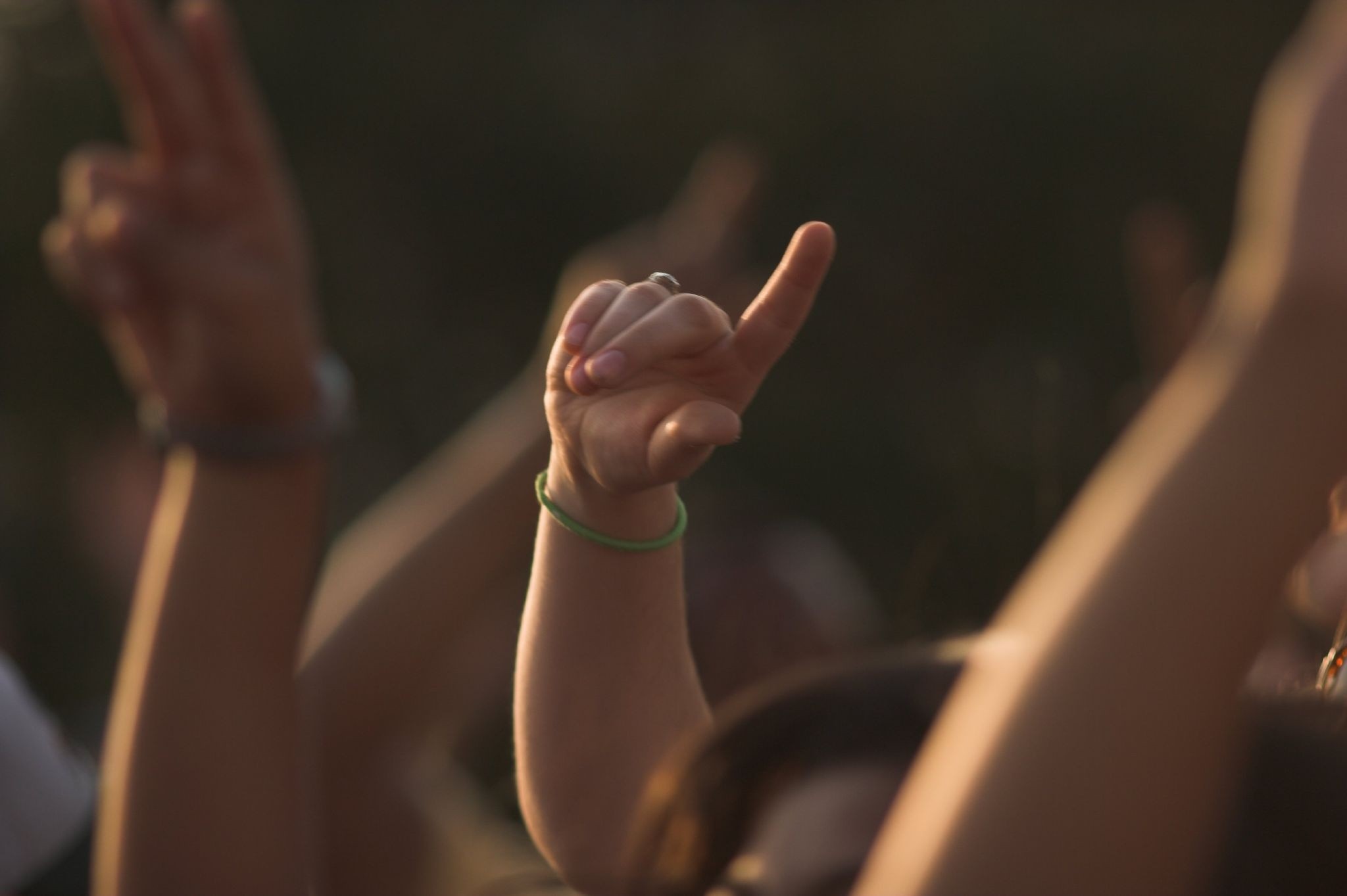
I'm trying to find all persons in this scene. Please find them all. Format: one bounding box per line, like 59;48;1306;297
0;0;1347;896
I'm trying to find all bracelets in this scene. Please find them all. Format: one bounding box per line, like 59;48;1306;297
532;470;688;552
